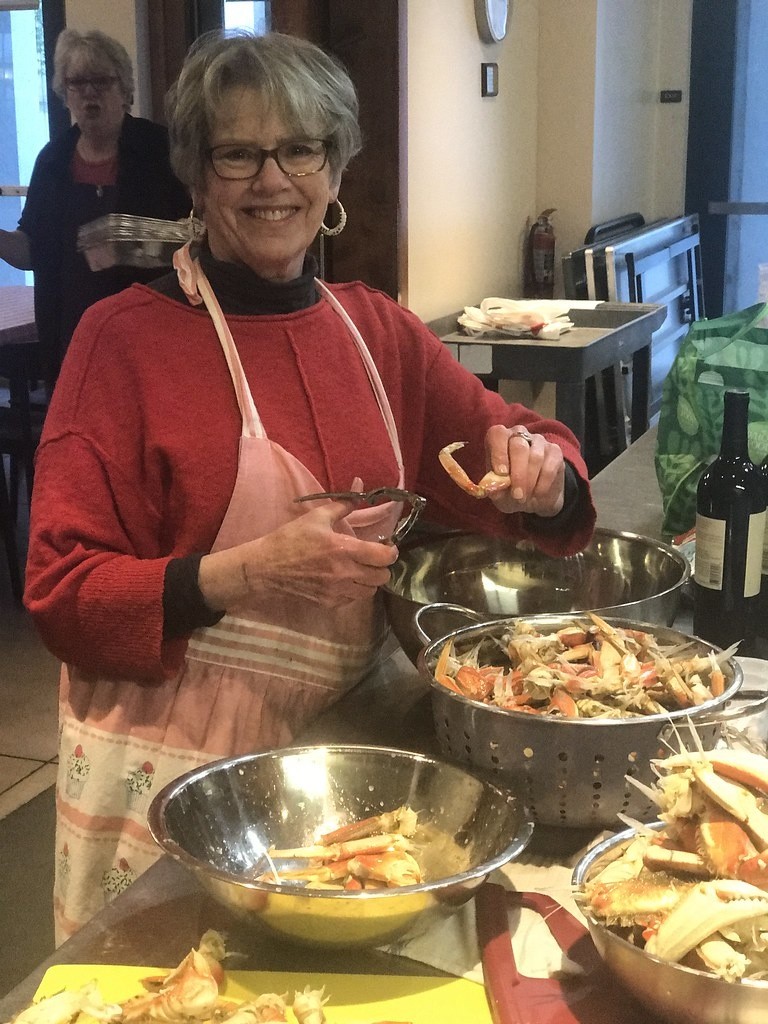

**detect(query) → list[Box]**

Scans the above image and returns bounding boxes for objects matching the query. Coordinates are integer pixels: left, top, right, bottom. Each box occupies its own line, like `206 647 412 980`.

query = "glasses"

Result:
200 138 337 180
62 73 120 92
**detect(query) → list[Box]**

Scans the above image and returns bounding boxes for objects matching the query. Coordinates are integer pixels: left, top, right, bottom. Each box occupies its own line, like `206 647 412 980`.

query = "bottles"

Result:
692 386 768 642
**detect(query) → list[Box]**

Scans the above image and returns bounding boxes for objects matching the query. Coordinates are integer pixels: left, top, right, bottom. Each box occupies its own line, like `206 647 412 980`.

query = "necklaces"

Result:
78 137 118 195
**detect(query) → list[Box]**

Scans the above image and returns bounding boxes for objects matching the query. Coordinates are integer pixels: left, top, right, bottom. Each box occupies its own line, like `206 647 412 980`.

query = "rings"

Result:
508 432 531 446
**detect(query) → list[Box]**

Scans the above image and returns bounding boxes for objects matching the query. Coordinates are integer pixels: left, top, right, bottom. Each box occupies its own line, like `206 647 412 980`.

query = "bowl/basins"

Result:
145 742 535 954
572 820 768 1024
378 523 692 672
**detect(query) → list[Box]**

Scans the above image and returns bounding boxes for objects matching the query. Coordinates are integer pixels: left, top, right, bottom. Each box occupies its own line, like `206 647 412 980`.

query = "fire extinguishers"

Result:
525 207 559 299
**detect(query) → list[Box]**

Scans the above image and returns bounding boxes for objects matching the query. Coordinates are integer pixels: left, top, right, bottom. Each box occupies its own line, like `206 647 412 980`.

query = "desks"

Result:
0 284 49 604
422 297 668 480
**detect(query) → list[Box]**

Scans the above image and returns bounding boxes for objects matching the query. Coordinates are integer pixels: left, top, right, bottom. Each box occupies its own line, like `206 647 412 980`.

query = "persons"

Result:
0 29 192 410
25 30 596 950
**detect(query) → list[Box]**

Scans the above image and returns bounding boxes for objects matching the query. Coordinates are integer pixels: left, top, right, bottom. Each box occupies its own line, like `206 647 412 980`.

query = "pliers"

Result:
292 488 424 542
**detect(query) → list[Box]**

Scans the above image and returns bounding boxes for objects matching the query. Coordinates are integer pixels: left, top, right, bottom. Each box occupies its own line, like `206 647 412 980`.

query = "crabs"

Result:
433 608 741 717
547 716 768 986
260 800 425 887
438 440 511 499
